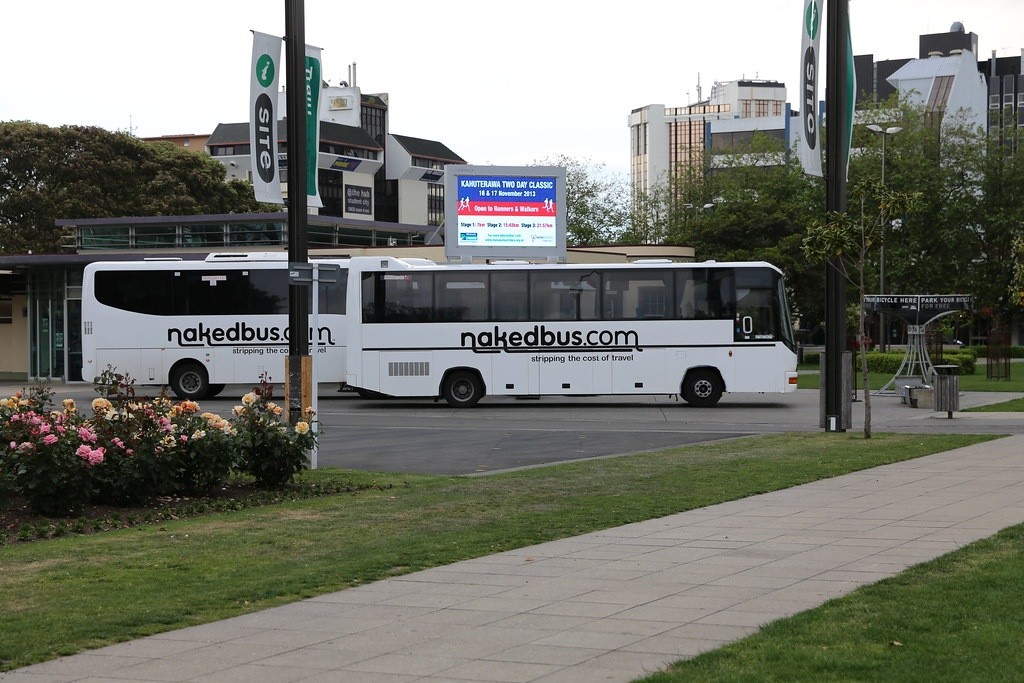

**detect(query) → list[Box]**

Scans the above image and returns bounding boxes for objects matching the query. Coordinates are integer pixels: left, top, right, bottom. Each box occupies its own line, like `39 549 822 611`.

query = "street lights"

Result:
865 124 905 352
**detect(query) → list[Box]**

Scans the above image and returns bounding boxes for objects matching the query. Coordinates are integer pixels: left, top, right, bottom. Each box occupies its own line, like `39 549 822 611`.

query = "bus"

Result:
82 252 802 409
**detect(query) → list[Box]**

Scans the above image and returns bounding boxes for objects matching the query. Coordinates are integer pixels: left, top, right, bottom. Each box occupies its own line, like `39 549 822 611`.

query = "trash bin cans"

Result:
798 346 803 364
819 352 853 429
933 366 960 419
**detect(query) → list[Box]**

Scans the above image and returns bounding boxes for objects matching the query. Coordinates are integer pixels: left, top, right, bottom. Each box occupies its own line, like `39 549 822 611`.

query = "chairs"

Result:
635 307 646 319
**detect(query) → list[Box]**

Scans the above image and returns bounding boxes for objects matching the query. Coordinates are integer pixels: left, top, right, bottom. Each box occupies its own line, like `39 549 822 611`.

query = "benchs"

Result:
904 386 934 410
